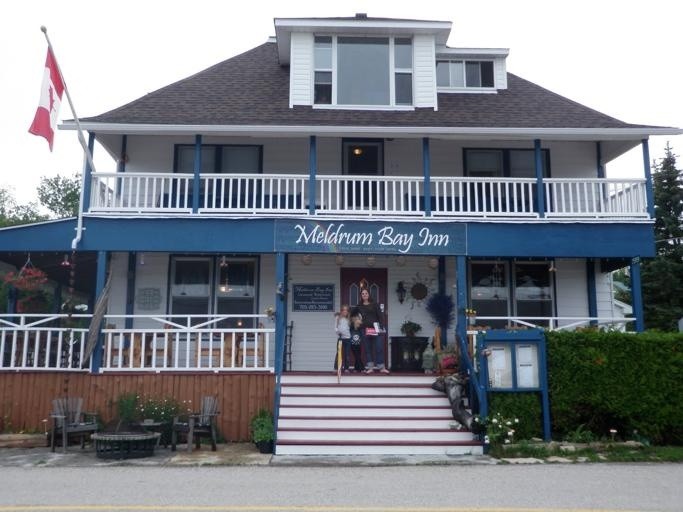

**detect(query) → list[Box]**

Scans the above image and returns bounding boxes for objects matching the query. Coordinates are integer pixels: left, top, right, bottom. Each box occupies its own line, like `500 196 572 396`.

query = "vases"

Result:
494 432 508 445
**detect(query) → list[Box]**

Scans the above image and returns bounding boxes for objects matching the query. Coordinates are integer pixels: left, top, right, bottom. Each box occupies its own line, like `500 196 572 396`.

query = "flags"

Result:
28 46 64 152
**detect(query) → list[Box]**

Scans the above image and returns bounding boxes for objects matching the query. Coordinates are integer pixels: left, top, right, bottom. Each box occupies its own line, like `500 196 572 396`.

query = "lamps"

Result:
396 281 406 304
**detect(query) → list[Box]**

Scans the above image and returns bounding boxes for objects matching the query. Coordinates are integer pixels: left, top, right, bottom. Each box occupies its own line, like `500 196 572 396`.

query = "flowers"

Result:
133 390 192 422
473 412 521 445
1 266 49 292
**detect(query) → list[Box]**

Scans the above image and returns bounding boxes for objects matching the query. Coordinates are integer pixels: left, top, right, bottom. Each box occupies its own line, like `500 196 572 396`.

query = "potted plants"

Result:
399 320 421 336
248 408 275 453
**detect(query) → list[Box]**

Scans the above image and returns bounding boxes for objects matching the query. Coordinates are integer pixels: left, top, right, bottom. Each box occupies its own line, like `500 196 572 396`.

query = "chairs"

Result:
104 323 268 368
47 397 99 454
169 394 221 452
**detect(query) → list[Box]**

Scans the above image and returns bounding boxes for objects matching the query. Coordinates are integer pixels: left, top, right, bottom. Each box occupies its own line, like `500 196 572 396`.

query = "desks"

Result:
87 430 161 459
388 335 429 370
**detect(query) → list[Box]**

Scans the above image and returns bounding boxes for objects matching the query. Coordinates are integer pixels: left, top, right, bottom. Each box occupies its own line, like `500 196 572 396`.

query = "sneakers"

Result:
333 365 391 376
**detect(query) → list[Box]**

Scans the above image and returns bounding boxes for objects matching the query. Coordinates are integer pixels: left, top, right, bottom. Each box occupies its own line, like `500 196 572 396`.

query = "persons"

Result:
335 285 390 374
